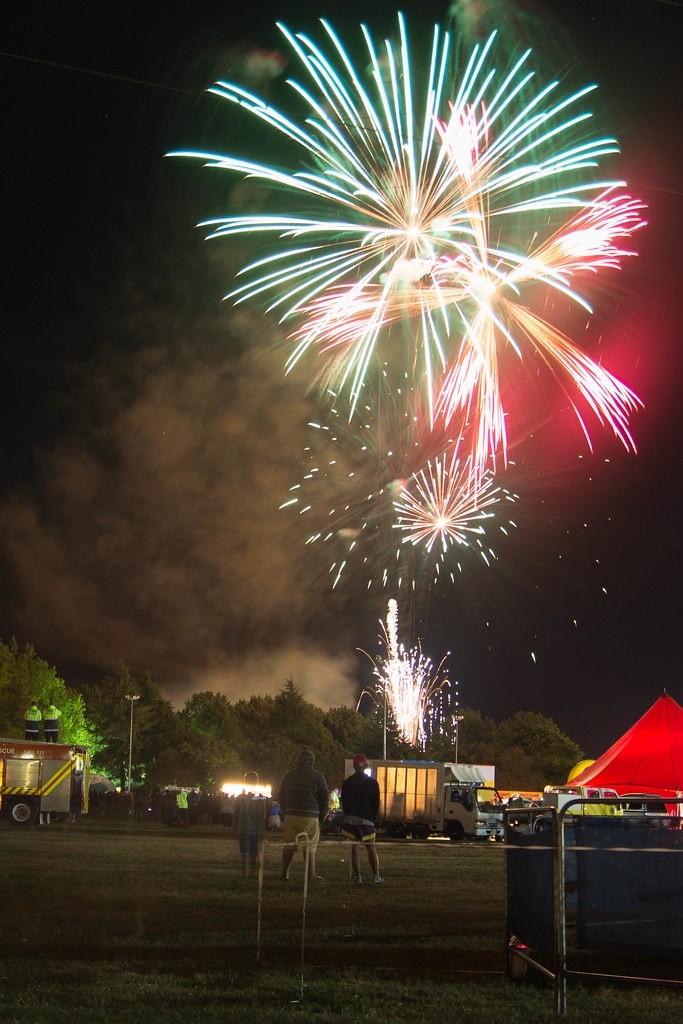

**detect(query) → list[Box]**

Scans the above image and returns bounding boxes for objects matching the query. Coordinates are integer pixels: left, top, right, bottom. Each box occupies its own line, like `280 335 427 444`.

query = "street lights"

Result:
124 693 141 794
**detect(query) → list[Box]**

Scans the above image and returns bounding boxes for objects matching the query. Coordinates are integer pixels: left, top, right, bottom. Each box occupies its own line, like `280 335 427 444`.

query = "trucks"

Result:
344 758 509 843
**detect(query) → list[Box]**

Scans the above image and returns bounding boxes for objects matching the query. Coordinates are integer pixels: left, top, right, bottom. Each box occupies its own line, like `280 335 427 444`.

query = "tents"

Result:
543 688 683 816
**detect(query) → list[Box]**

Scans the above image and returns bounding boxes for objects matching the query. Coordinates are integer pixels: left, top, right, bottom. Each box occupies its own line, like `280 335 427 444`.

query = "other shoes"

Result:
352 875 362 882
373 876 384 883
280 878 289 881
310 876 323 881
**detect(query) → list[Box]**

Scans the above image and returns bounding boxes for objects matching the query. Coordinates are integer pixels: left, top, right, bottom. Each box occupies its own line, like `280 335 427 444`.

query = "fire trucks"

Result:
0 738 91 826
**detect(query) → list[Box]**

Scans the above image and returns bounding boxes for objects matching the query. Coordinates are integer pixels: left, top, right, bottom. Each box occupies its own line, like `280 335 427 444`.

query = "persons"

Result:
278 749 329 880
42 701 62 743
24 701 42 742
232 771 265 882
451 791 465 804
70 771 343 835
339 754 384 884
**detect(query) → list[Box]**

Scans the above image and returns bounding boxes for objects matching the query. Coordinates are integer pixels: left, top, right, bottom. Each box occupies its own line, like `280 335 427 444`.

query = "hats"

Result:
297 751 314 765
353 754 367 766
